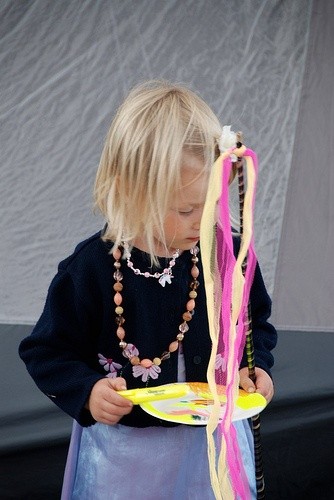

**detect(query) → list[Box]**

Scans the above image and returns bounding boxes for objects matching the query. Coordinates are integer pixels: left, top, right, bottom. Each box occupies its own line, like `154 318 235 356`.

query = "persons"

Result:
18 76 278 500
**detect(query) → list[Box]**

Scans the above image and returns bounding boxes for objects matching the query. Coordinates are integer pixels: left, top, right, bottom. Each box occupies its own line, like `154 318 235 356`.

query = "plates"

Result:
139 382 268 426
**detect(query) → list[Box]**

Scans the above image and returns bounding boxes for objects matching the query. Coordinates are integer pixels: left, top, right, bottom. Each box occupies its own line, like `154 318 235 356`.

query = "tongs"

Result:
116 382 190 404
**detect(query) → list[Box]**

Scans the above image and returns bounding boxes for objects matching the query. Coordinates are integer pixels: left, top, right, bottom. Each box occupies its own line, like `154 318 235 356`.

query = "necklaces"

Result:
113 243 199 382
123 242 180 287
